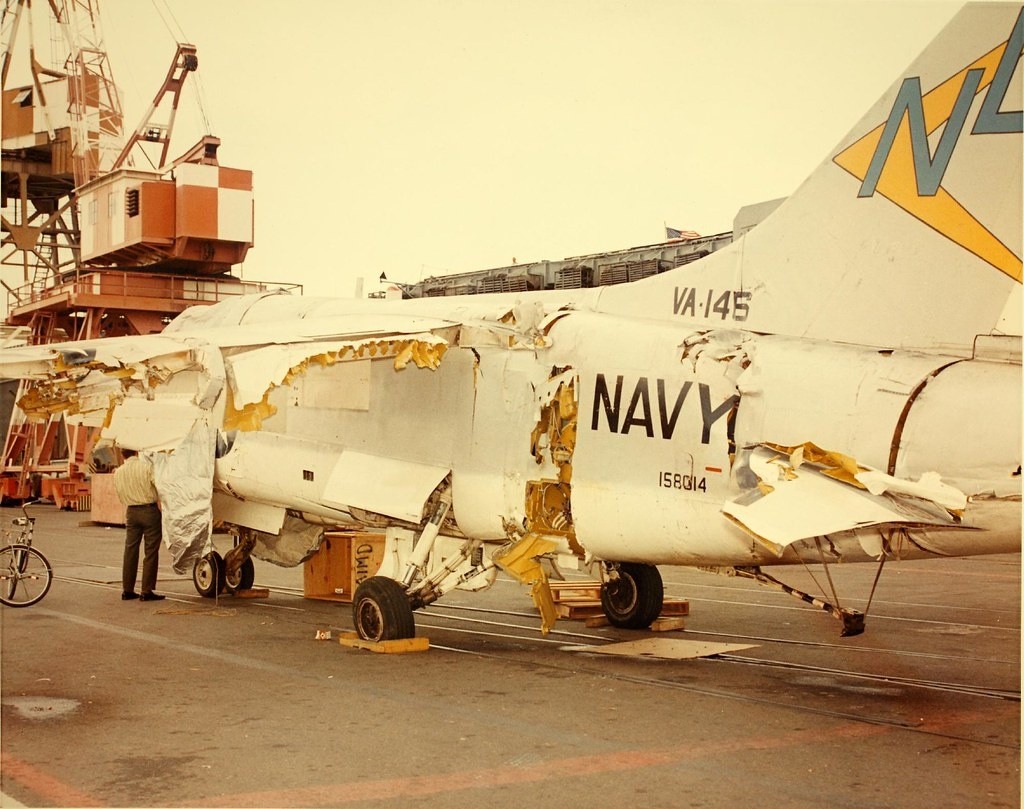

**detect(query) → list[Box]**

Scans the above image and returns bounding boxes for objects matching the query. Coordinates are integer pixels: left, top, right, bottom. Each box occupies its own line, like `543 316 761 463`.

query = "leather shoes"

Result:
122 591 165 601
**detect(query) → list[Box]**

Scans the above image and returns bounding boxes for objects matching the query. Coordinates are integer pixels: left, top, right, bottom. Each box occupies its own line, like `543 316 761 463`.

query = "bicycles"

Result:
0 498 53 608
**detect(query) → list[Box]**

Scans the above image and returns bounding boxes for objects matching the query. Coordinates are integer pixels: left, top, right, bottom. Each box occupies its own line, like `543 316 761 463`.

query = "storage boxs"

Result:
303 532 387 603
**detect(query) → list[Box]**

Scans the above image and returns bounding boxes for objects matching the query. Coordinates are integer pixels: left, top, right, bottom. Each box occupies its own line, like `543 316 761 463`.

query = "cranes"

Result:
1 0 304 499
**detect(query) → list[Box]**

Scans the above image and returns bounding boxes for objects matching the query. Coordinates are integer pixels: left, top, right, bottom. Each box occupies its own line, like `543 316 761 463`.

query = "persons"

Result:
114 448 166 602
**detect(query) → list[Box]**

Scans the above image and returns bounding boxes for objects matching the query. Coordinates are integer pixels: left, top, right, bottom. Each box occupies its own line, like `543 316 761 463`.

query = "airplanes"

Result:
0 1 1024 642
